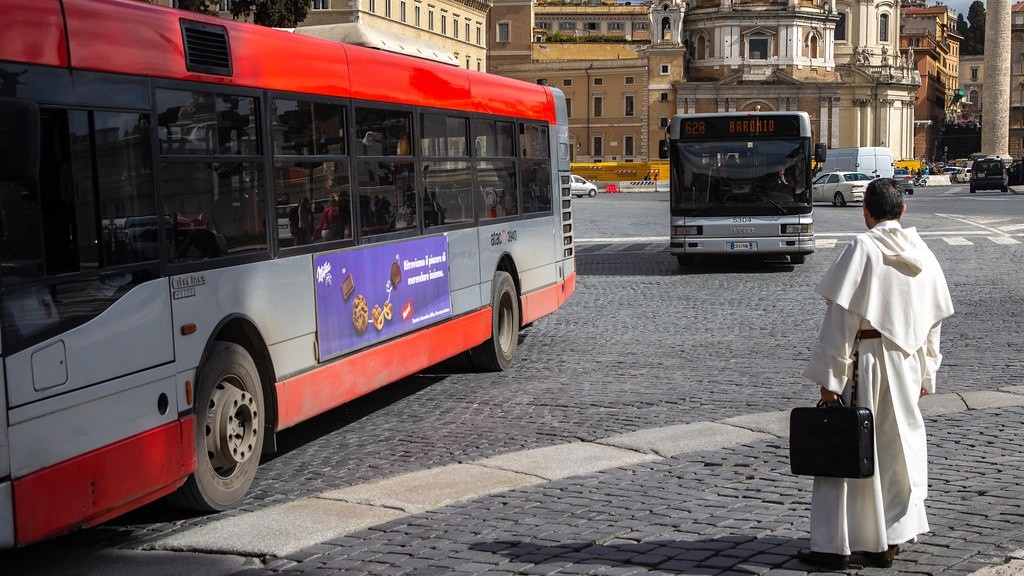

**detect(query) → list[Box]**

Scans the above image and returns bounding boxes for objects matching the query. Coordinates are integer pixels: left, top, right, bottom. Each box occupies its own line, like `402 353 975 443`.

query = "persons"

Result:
769 165 795 185
897 166 929 179
287 192 353 246
786 178 954 567
644 171 651 180
654 174 658 180
853 45 913 68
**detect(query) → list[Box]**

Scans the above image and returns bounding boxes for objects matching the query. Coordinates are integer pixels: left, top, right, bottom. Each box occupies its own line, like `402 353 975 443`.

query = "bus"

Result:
0 1 576 551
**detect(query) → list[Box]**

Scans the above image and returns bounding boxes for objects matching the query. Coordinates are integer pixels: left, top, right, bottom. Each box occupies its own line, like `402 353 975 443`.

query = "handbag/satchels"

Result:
789 394 875 478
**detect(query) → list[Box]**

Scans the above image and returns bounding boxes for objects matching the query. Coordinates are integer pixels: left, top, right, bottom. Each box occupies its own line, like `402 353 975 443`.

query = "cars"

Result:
276 203 300 239
813 171 874 207
570 174 598 198
934 161 972 185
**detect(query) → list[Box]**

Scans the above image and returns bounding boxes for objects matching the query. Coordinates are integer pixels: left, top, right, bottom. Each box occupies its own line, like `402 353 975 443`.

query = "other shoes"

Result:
863 545 900 568
799 548 850 570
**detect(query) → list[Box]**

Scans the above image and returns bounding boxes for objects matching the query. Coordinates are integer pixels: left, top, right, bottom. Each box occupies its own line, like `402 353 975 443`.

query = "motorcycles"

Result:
913 175 927 188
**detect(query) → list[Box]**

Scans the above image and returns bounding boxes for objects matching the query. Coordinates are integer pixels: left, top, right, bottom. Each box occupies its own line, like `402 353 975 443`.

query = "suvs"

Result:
969 157 1008 194
893 167 914 196
658 110 827 266
423 201 447 224
118 216 217 263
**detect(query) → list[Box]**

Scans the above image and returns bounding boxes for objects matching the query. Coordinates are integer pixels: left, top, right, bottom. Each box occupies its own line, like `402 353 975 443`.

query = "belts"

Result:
856 329 881 339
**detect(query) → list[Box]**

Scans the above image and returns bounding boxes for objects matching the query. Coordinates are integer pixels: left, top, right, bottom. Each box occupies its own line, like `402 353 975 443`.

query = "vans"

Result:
812 147 895 179
